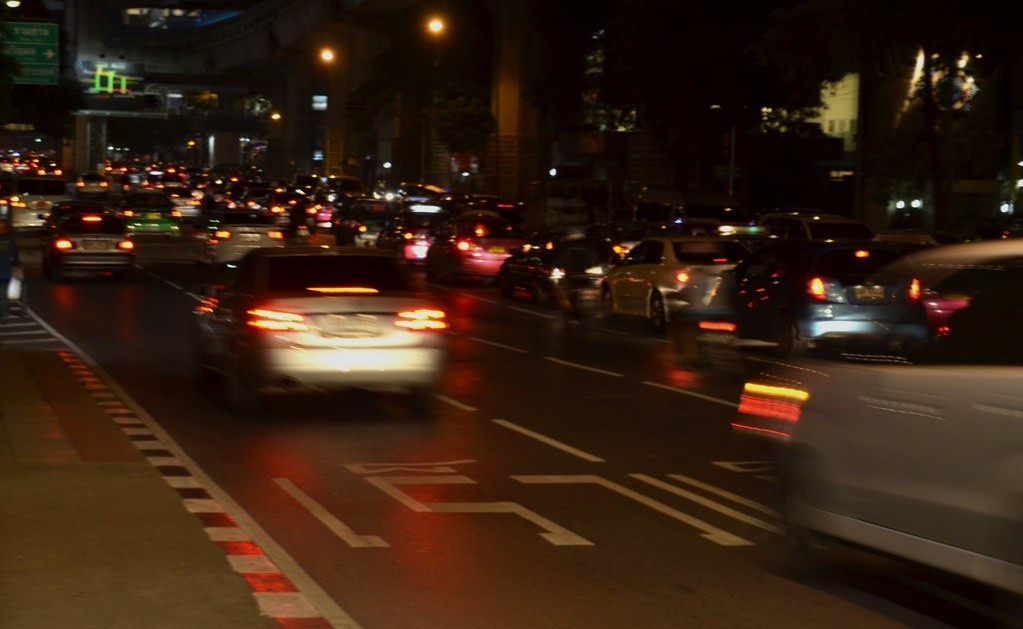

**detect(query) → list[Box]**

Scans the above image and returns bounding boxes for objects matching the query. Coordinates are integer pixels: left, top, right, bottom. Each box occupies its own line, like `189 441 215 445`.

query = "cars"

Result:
771 245 929 373
600 234 749 341
193 246 445 420
0 136 930 320
899 242 1021 360
753 243 1020 602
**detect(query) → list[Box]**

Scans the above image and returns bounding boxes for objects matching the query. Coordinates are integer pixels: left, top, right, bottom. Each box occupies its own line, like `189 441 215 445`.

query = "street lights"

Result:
426 15 449 186
319 45 335 175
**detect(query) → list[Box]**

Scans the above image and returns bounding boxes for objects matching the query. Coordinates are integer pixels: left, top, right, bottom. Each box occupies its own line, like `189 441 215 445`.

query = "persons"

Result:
0 218 23 317
